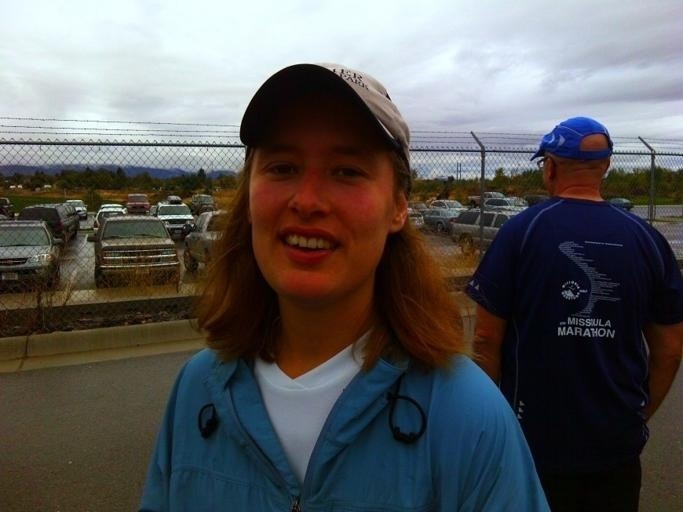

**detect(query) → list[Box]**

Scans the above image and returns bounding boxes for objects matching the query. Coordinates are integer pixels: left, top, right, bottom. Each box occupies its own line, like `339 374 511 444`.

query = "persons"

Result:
471 116 681 510
137 65 549 510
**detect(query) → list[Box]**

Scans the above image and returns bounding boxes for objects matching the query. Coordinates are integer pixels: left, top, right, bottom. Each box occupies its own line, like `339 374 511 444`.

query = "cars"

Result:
0 218 63 292
403 191 548 235
92 204 128 234
607 198 634 211
191 195 215 211
0 198 16 220
66 200 88 220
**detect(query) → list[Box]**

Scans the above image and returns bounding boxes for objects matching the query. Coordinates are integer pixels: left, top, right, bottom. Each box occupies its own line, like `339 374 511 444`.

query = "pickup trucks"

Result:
182 209 229 272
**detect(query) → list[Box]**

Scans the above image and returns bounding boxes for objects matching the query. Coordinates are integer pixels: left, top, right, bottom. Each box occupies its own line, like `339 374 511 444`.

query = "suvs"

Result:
127 193 151 214
17 203 81 244
156 196 196 238
450 210 521 254
86 214 181 288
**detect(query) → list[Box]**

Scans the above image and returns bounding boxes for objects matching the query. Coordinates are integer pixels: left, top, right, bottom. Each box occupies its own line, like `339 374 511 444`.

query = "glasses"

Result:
535 156 555 170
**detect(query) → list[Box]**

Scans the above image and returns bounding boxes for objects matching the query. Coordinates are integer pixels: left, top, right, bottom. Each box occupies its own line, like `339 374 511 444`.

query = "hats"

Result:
240 61 411 168
530 116 614 160
